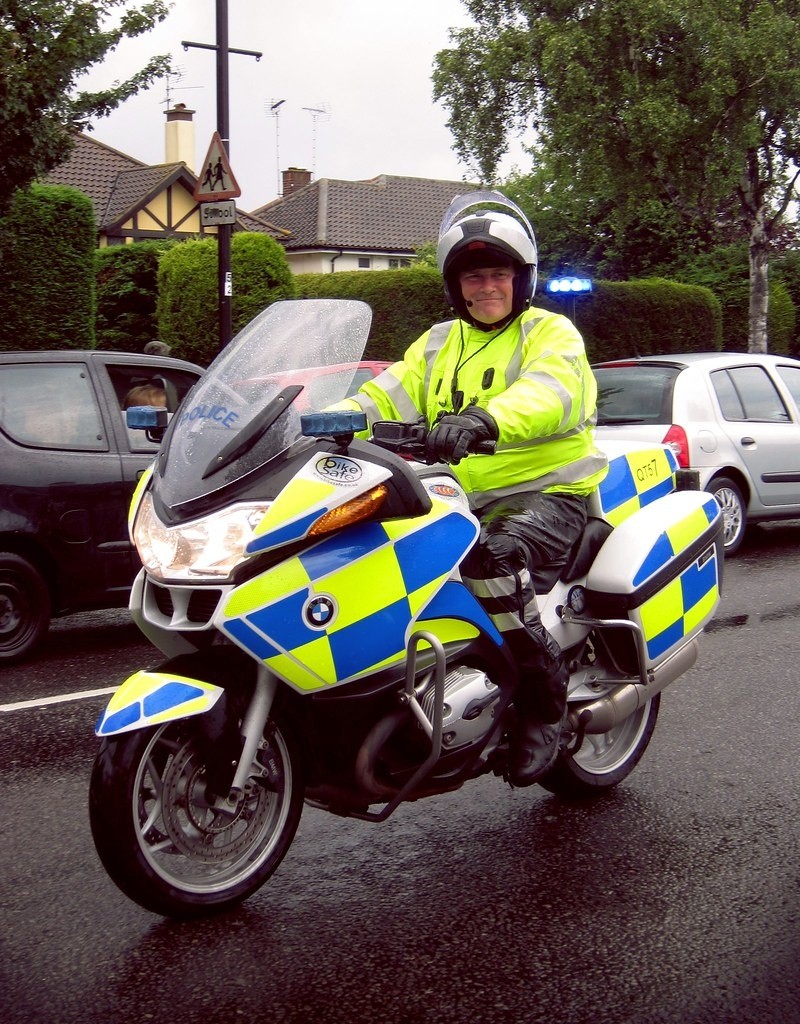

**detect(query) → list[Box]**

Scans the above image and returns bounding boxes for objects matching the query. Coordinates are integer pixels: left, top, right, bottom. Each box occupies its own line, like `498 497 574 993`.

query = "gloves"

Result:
424 405 500 467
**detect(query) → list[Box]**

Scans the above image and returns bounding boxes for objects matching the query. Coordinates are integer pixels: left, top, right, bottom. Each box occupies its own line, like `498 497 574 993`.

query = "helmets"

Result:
436 189 538 312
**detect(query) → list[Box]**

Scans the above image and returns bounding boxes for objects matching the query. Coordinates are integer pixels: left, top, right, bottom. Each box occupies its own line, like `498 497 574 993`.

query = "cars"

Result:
0 350 250 668
589 353 800 558
227 361 395 415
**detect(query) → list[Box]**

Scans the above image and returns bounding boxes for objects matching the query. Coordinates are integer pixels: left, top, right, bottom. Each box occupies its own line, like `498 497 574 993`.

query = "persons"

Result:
120 340 171 411
299 207 602 787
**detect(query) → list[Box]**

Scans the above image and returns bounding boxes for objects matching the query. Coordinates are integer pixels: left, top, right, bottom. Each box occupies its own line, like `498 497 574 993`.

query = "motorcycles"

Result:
87 276 726 920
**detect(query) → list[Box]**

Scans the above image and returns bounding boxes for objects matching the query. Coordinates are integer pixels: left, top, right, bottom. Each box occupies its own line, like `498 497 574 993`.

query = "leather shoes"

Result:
506 703 568 788
